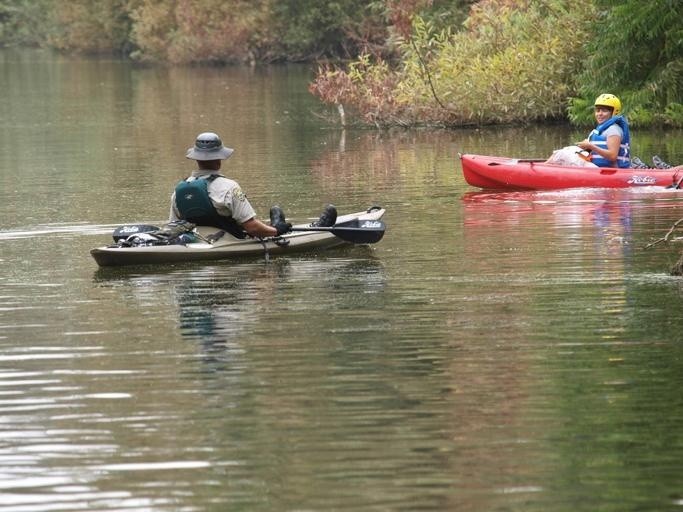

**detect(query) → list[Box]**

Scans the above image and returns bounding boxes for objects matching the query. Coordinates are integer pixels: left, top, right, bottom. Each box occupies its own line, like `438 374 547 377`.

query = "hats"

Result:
185 132 235 162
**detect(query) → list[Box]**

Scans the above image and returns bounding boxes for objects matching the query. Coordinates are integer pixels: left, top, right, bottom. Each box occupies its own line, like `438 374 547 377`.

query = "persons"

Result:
548 93 630 168
168 132 337 248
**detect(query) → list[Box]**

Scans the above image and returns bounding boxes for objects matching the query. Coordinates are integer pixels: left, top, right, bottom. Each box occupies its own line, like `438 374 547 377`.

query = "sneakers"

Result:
309 204 338 228
270 205 286 228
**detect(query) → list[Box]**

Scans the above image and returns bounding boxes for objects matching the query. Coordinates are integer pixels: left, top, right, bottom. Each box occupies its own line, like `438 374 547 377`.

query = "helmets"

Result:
594 93 622 119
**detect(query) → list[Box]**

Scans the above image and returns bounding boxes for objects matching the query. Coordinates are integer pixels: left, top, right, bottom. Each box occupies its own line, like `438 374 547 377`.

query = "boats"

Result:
458 153 683 191
90 205 386 272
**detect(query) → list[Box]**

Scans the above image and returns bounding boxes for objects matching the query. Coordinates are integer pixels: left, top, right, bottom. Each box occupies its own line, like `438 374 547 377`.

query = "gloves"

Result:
275 222 292 235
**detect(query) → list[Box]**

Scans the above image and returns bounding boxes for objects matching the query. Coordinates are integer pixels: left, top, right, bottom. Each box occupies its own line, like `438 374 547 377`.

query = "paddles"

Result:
289 218 386 243
113 224 161 244
